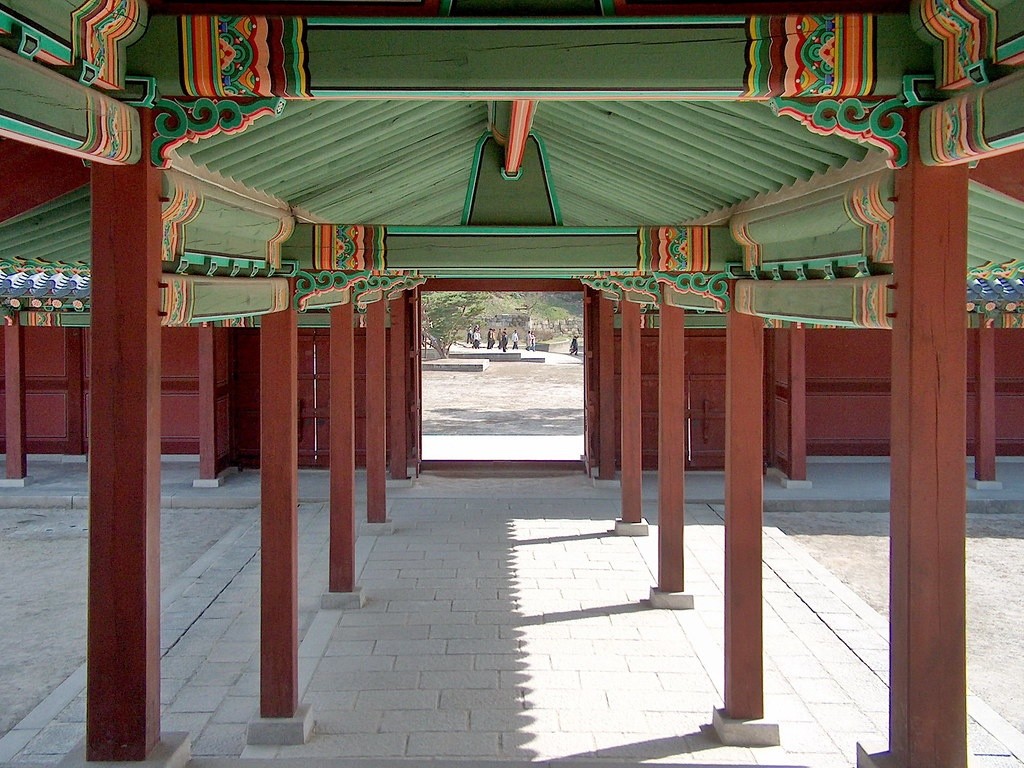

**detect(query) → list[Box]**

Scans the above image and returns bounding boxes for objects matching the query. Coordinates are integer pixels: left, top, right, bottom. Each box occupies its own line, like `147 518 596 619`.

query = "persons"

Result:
569 334 580 356
466 323 537 352
